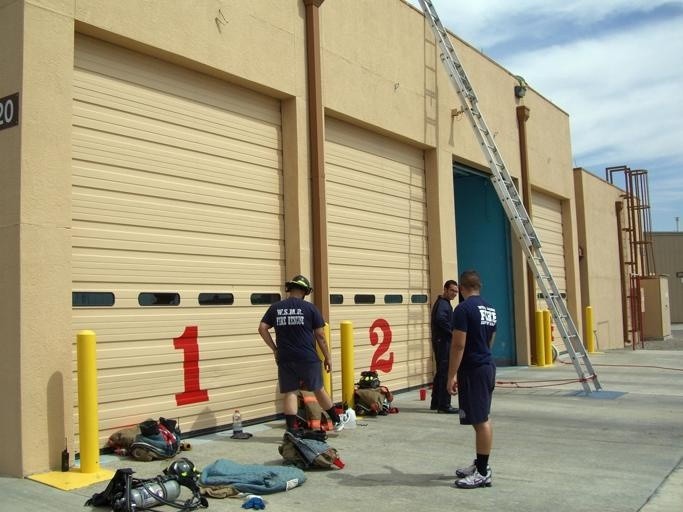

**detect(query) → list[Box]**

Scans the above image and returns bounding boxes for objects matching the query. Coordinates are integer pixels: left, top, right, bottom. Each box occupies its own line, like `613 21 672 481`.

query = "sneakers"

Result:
333 413 350 432
455 468 491 488
456 459 489 478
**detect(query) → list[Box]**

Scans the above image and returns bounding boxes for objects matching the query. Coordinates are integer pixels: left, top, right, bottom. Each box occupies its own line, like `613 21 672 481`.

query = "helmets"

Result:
285 275 310 295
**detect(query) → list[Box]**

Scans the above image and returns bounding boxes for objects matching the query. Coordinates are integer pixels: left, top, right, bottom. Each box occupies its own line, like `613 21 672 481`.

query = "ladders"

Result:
417 0 602 395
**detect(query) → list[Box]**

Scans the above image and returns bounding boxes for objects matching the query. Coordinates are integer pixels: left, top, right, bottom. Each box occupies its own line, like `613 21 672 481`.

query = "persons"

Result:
258 274 351 434
430 280 460 413
447 270 497 489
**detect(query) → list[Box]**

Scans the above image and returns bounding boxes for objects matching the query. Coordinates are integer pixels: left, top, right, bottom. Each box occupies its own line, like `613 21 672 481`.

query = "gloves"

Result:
242 495 265 510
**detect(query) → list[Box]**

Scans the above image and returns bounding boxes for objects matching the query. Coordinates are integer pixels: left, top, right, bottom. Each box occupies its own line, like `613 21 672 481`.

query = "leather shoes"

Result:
431 404 460 413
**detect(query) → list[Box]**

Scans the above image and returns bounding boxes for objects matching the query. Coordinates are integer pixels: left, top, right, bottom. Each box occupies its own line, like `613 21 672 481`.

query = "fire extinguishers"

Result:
551 324 555 341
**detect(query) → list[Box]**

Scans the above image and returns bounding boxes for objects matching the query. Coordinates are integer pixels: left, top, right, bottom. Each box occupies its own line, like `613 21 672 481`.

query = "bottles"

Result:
419 385 426 400
62 450 69 472
231 409 242 434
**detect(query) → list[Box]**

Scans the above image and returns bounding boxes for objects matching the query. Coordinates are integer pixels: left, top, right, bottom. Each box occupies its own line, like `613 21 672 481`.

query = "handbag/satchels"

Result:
279 431 344 471
